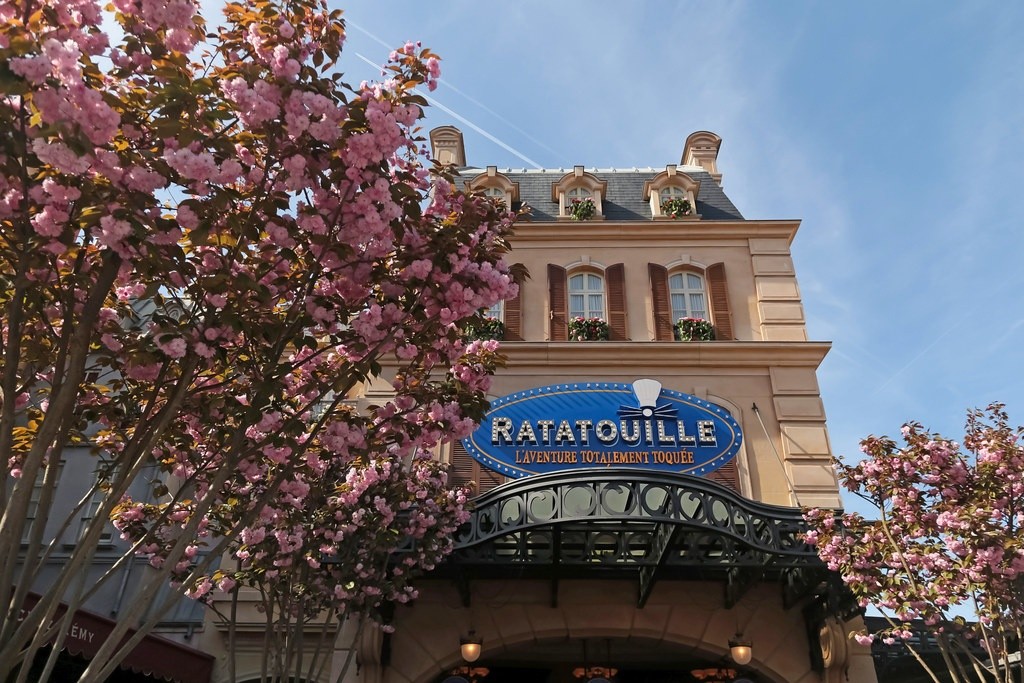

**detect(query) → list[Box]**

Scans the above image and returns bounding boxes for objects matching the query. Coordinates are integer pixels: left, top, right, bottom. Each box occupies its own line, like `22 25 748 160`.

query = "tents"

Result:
4 584 216 683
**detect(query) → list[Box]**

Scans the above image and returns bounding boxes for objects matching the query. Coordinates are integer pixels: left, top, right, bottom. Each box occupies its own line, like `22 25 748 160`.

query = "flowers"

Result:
569 316 608 342
470 317 503 342
569 197 595 221
678 317 713 342
662 197 691 219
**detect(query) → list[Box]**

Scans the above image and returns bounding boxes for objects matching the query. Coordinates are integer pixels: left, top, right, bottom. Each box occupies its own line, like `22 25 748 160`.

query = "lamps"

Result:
460 579 483 662
728 569 753 665
